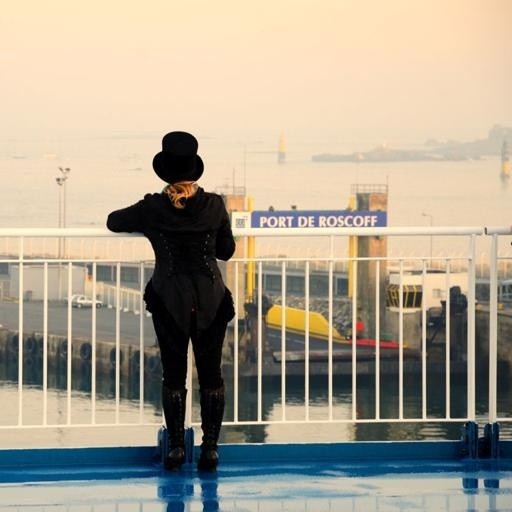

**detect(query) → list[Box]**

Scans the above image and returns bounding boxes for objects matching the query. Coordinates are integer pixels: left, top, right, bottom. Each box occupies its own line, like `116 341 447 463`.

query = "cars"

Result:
63 294 103 309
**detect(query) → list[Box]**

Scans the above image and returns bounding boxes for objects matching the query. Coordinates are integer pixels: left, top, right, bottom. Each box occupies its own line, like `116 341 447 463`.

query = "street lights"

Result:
54 166 71 258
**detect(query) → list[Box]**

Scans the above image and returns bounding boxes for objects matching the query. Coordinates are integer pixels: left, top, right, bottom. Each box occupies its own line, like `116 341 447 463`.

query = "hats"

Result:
153 131 203 185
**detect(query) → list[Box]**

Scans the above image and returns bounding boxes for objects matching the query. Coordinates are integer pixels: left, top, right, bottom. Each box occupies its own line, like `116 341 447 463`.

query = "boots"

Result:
160 386 187 473
197 386 225 472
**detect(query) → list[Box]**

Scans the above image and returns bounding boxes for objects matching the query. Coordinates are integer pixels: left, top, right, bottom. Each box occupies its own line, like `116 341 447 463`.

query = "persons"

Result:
106 132 237 474
